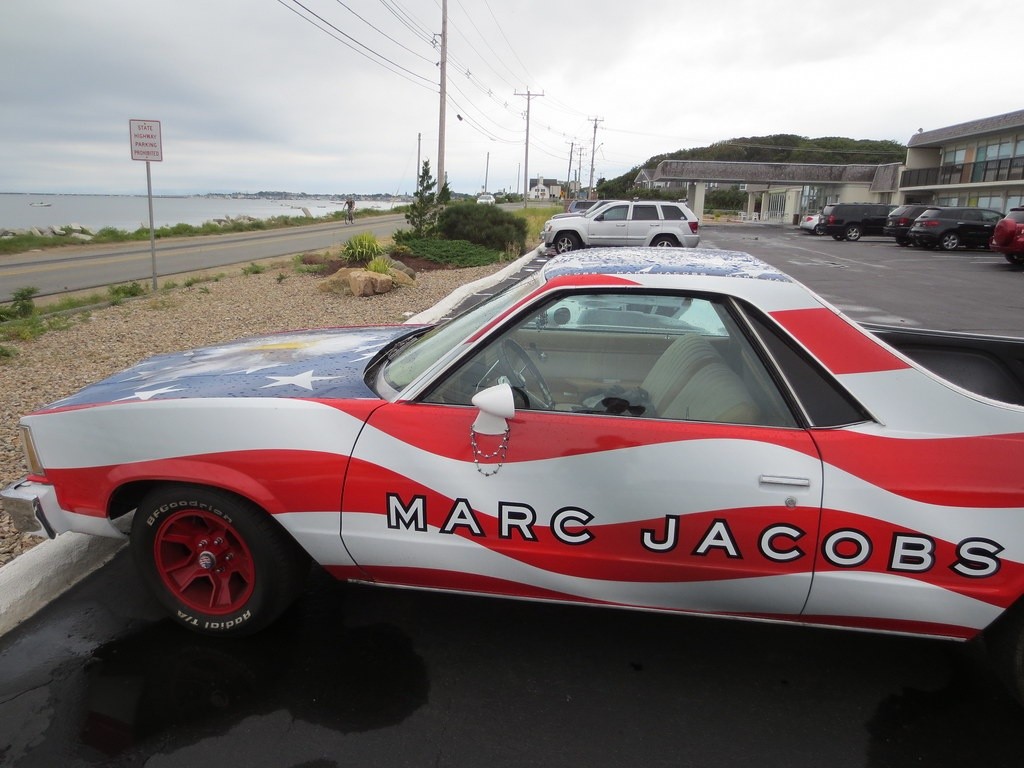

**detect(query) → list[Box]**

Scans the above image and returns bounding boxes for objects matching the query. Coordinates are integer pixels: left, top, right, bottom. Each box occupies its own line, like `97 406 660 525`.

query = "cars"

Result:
475 195 497 206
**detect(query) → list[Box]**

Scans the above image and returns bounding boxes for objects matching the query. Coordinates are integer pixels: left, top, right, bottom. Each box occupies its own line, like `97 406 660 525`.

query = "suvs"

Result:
884 204 1005 251
541 198 700 254
988 204 1024 270
799 202 899 241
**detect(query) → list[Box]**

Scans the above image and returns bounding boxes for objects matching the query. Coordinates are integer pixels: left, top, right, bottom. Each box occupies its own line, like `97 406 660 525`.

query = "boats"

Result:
29 201 52 207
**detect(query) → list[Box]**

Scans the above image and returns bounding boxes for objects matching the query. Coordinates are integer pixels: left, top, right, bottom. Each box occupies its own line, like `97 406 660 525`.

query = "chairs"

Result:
640 332 762 424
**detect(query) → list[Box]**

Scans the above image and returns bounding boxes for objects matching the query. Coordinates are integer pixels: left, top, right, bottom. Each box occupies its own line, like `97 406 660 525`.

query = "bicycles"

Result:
344 209 355 225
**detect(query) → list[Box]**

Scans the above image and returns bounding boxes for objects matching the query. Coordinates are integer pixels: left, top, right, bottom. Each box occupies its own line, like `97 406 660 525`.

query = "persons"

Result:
343 196 355 221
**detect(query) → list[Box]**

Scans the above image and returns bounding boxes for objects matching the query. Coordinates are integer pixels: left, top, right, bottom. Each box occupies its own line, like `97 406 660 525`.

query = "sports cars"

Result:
1 246 1024 651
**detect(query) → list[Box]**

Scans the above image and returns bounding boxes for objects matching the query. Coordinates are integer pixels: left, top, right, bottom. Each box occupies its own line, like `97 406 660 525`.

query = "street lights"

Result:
588 143 604 200
